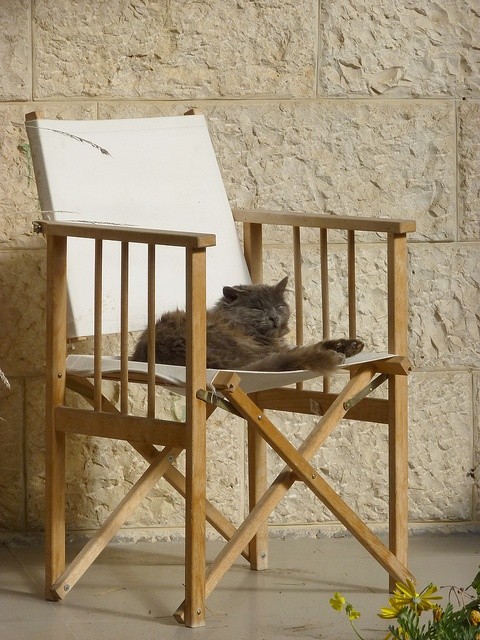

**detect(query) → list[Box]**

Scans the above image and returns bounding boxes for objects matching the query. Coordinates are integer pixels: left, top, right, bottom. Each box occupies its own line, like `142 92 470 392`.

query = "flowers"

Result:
330 581 479 639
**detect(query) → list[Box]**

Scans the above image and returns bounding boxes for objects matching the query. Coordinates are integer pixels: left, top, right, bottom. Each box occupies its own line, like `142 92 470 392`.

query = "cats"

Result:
129 276 364 374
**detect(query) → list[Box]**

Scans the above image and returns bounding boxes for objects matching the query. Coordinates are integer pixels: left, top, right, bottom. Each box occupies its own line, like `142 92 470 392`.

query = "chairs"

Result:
26 103 417 629
